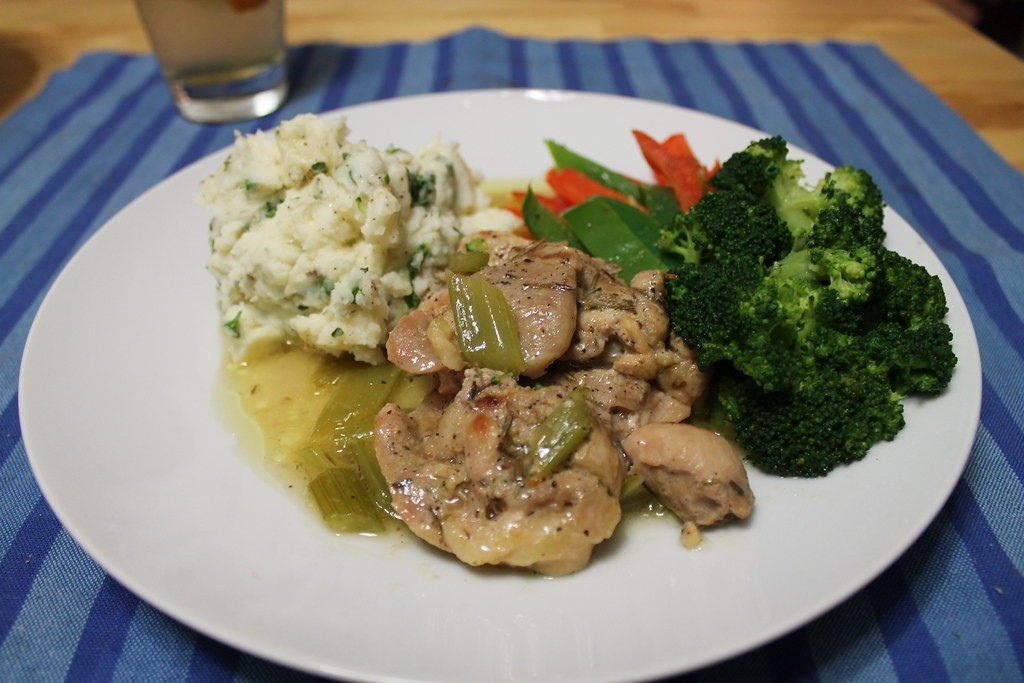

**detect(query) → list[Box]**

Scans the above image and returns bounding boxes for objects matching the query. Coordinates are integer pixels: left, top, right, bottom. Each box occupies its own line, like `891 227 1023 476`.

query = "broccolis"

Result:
653 134 958 479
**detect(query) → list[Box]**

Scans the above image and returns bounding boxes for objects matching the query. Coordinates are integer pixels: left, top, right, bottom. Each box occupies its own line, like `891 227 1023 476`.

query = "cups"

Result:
129 0 291 124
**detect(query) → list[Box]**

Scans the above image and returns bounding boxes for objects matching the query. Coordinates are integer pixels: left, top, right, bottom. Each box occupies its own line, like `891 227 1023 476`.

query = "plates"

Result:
17 87 983 683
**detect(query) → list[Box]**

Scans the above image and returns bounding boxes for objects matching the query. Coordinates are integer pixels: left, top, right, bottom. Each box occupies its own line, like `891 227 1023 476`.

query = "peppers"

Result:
507 129 719 283
